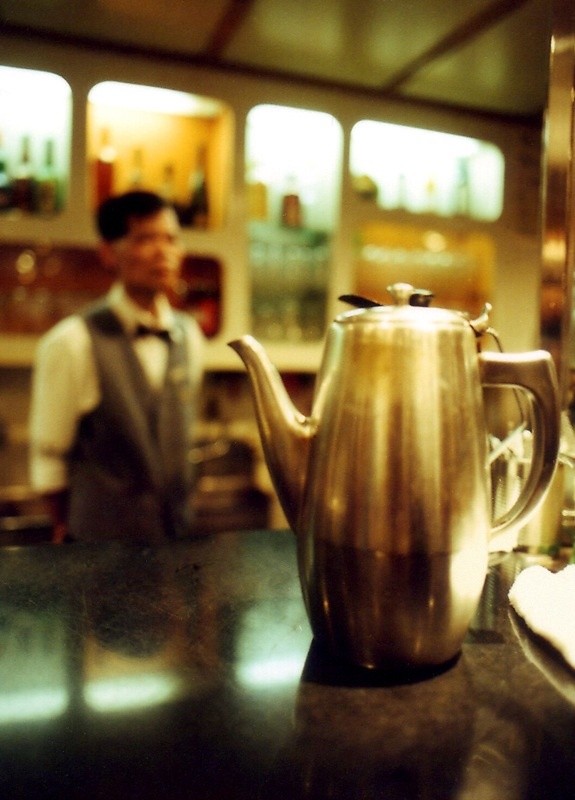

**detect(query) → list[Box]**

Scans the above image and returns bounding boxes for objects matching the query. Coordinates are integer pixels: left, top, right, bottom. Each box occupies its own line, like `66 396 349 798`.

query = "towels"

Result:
507 564 575 669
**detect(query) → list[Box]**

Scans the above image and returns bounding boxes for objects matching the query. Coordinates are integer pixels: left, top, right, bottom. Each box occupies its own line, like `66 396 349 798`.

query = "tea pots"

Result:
226 282 563 681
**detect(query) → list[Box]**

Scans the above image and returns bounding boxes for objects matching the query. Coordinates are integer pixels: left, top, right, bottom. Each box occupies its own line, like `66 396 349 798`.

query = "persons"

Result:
27 190 207 547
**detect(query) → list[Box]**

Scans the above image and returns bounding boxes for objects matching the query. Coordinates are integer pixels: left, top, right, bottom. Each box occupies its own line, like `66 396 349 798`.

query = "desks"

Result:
0 520 575 800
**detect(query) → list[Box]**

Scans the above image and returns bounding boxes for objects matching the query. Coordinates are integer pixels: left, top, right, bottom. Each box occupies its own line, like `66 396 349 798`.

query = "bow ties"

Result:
135 323 172 345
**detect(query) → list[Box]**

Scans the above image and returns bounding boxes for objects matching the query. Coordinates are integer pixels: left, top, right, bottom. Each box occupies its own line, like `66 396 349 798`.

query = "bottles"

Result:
0 134 211 228
